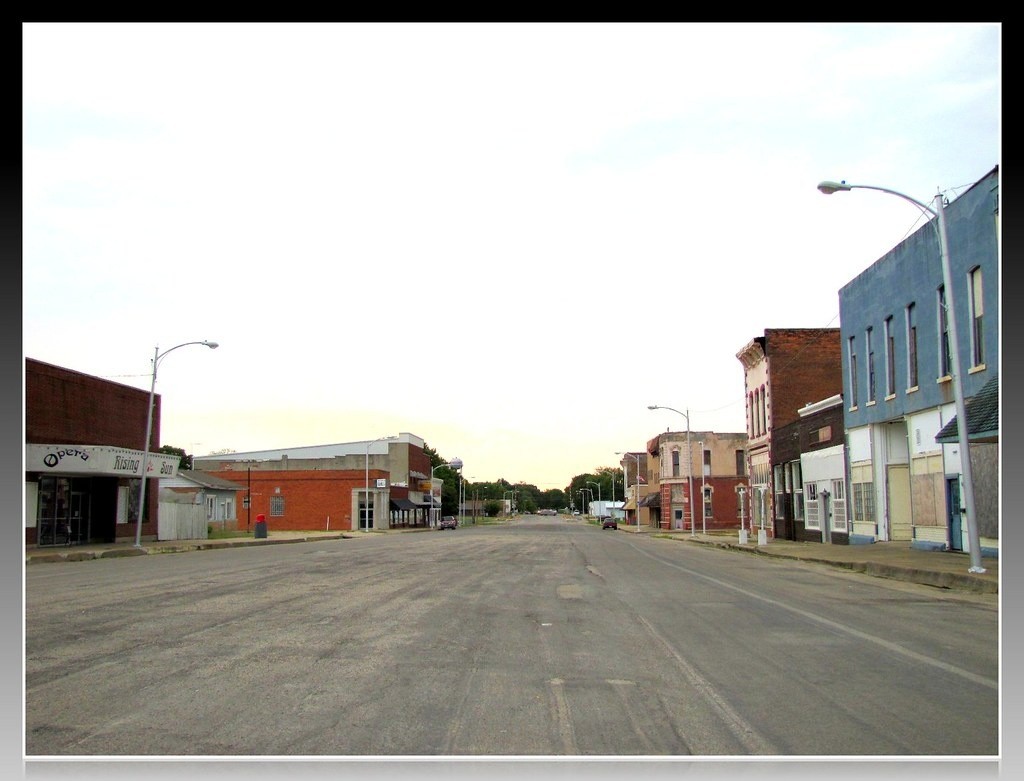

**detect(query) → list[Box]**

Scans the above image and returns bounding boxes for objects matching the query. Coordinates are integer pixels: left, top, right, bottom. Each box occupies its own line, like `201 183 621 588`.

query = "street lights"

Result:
459 477 475 526
580 489 595 519
615 451 640 529
135 341 219 545
648 406 696 538
365 435 395 532
431 463 462 530
576 491 584 515
817 182 982 573
587 481 601 525
597 470 614 518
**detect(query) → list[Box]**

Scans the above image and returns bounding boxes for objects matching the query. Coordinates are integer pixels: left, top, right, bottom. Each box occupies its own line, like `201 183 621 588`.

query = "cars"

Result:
603 518 617 530
538 509 557 516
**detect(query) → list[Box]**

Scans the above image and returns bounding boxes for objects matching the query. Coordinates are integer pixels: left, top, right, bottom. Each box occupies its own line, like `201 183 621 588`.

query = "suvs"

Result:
574 510 579 515
441 516 456 530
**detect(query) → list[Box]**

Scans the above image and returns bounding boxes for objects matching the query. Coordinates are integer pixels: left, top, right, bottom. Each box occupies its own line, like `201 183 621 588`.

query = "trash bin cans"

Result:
254 514 268 539
